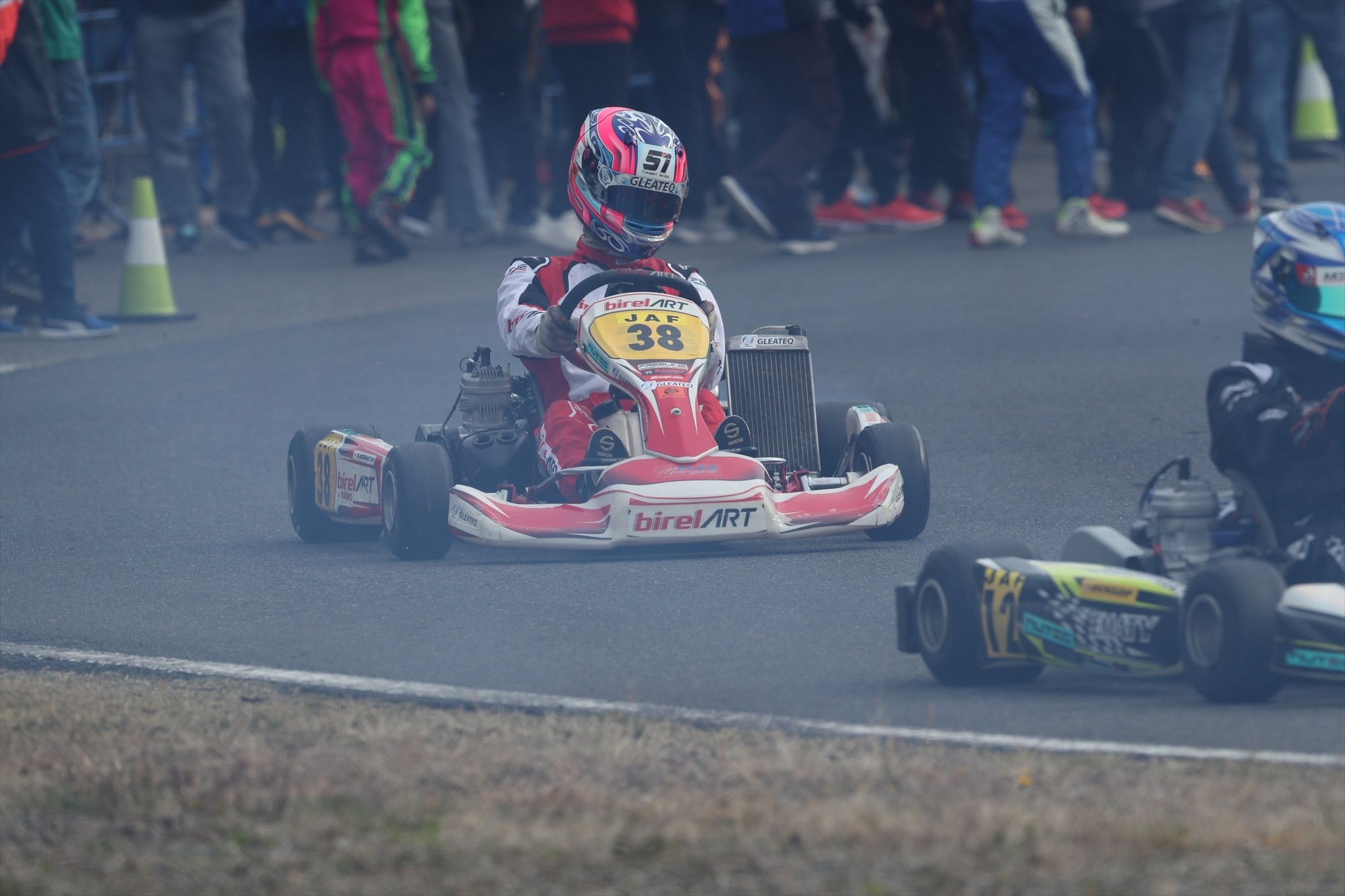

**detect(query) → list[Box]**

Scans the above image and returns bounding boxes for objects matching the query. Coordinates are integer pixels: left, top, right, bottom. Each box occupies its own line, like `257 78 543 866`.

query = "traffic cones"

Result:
92 177 200 325
1286 33 1341 158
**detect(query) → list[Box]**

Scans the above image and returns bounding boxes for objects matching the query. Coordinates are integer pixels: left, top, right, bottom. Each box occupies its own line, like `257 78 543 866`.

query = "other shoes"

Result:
508 207 586 253
906 190 963 218
1259 187 1314 209
37 307 119 338
969 205 1024 250
674 213 736 244
176 222 198 246
255 208 323 242
777 224 836 255
1235 201 1262 225
1088 192 1129 220
0 319 29 339
587 428 628 485
1054 196 1131 239
811 191 949 232
356 209 410 262
714 414 753 458
1155 194 1222 235
957 192 1025 229
219 212 259 243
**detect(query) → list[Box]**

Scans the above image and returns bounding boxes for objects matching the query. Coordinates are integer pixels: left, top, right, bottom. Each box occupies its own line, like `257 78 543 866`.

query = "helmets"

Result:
1244 200 1344 362
565 107 688 259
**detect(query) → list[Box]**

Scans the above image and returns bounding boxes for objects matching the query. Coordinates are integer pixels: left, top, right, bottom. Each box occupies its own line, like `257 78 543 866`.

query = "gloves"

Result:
534 302 579 358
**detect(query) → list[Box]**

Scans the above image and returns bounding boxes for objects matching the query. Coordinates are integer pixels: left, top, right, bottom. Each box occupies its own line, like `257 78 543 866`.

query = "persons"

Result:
0 0 1345 338
1207 202 1345 586
497 106 752 505
